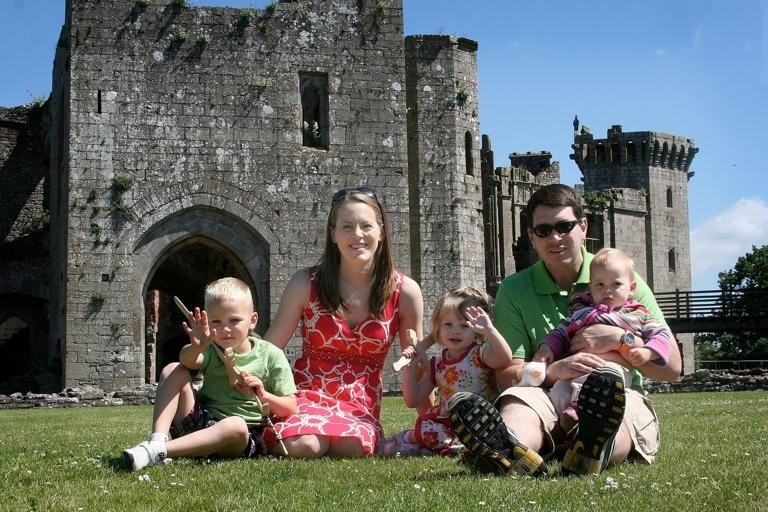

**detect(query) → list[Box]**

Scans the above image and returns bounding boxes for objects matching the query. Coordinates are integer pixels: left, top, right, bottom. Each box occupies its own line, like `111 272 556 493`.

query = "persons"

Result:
382 285 515 460
263 188 434 460
532 247 672 433
445 183 684 481
120 276 297 472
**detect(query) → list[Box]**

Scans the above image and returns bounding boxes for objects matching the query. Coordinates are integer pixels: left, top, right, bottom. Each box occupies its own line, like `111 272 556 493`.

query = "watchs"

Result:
617 328 636 354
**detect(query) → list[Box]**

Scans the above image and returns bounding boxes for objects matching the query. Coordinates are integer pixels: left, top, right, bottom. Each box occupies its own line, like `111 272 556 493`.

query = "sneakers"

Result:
556 364 625 477
120 433 168 473
384 429 420 456
446 392 548 478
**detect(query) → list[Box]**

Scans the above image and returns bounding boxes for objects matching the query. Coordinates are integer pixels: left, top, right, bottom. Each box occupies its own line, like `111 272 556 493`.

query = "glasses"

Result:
332 187 380 200
531 218 582 239
